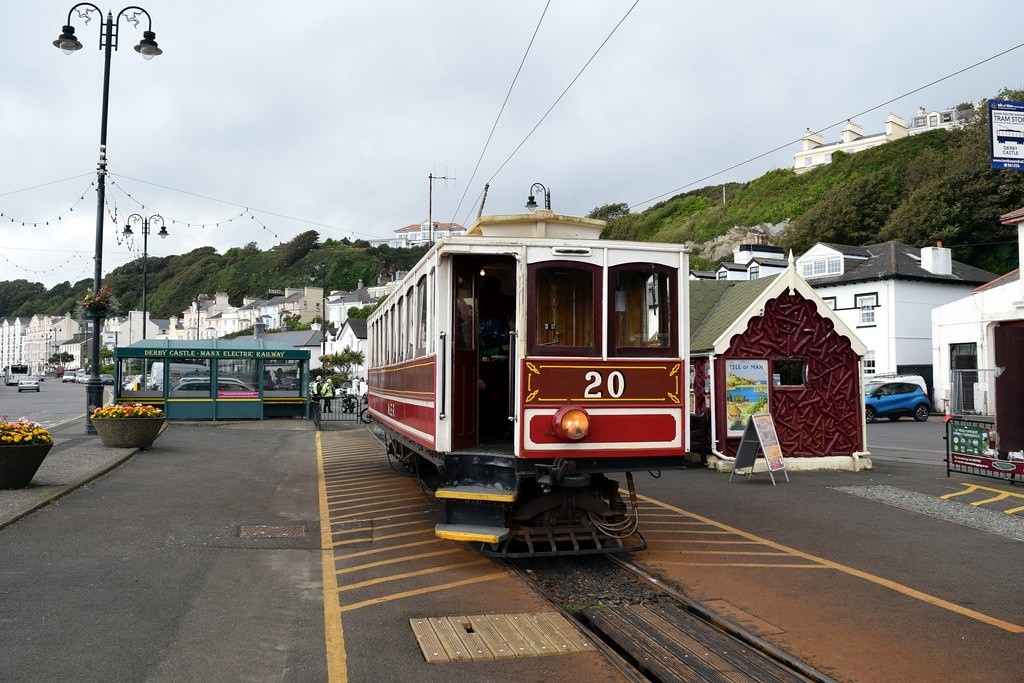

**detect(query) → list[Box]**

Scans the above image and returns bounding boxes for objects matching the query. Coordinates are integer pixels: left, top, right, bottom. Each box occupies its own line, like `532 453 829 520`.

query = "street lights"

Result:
309 263 327 383
524 181 552 212
189 299 200 340
122 214 170 388
49 325 63 353
122 312 132 345
41 332 52 369
80 320 88 375
50 2 164 435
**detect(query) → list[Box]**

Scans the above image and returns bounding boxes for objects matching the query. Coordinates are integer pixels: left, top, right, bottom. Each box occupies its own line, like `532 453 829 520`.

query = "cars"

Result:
61 362 257 396
17 376 41 392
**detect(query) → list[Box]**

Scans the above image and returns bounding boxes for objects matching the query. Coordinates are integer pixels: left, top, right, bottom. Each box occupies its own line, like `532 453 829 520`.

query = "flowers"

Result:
82 285 113 311
0 416 52 442
90 400 165 419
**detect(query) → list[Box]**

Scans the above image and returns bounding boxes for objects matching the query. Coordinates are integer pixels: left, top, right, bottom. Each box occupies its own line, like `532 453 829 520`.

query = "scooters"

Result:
360 407 372 424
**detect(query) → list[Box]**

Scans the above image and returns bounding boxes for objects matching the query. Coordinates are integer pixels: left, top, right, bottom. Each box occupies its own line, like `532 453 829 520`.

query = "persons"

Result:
322 379 334 413
314 376 323 402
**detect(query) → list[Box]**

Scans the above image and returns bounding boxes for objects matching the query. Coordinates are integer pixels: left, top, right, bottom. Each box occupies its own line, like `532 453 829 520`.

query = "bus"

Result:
2 364 31 385
365 234 694 562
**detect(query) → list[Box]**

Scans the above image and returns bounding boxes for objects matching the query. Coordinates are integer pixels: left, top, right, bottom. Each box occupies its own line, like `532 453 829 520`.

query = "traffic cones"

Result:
942 401 956 423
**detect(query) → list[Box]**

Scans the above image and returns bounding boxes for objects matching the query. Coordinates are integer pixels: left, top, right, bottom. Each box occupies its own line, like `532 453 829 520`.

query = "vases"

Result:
87 308 108 319
89 417 166 447
0 442 54 491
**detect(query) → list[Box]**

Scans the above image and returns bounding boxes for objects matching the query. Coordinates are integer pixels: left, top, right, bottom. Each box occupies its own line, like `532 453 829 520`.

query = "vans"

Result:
868 372 928 395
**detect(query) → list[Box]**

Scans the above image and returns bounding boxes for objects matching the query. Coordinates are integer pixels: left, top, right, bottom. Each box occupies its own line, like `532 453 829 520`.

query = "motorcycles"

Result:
338 382 356 414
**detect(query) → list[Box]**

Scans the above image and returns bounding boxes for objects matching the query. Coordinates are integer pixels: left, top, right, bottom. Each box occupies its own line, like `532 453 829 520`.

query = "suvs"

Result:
863 382 932 425
